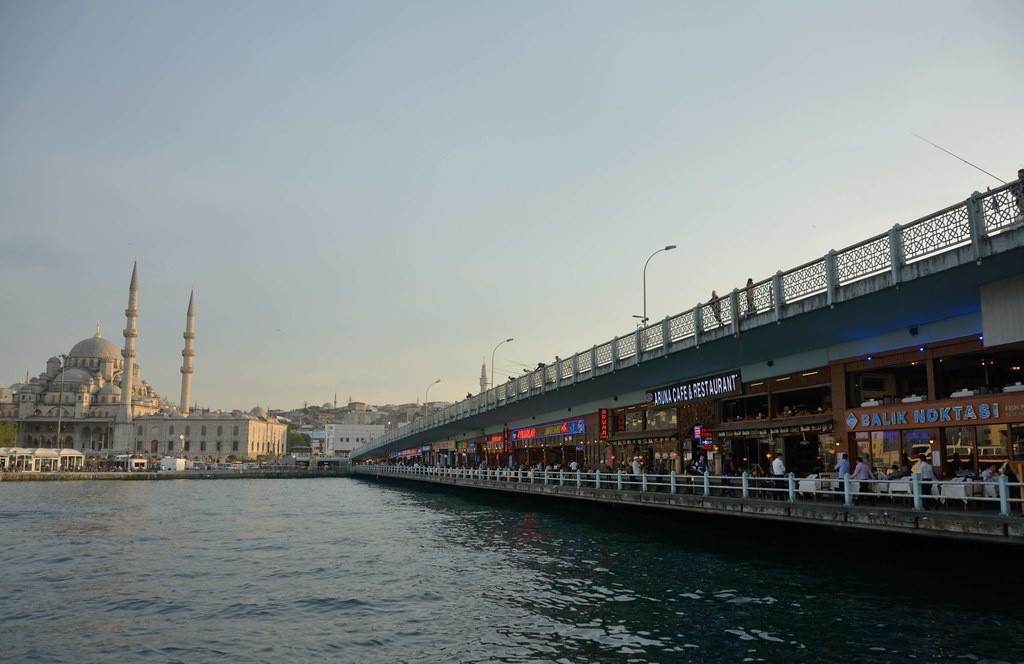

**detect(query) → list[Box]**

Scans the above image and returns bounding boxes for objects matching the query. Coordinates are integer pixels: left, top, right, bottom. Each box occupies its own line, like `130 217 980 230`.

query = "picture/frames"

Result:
862 378 886 391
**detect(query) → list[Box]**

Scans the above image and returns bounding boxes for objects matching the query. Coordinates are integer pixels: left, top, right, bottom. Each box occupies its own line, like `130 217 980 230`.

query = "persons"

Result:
386 458 703 497
693 452 999 500
639 327 650 351
708 290 725 325
507 361 549 395
745 278 757 313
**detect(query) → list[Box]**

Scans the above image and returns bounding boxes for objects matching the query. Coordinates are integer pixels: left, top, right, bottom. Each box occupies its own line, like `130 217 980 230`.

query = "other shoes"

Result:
935 505 941 510
924 506 930 511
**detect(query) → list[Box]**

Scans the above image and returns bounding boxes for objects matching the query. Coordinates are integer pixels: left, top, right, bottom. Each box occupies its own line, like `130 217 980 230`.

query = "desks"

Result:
534 473 728 497
871 483 889 507
940 480 983 512
733 419 742 421
985 486 1000 513
798 481 839 502
860 401 879 407
950 391 975 398
1002 385 1024 393
901 396 922 404
888 483 913 509
851 482 871 506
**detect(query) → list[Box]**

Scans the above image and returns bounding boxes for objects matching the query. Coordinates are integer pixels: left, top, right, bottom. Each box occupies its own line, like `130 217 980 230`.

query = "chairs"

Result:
795 410 804 416
968 477 984 511
727 417 736 422
817 474 830 501
776 412 783 418
742 416 750 420
979 383 990 395
750 415 756 420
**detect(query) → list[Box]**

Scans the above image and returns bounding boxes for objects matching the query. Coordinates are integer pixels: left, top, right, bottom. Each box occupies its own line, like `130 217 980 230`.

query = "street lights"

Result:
425 379 441 417
491 338 515 389
644 245 677 328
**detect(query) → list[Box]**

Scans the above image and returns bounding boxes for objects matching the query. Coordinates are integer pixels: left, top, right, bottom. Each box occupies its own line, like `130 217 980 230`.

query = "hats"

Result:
634 456 638 460
